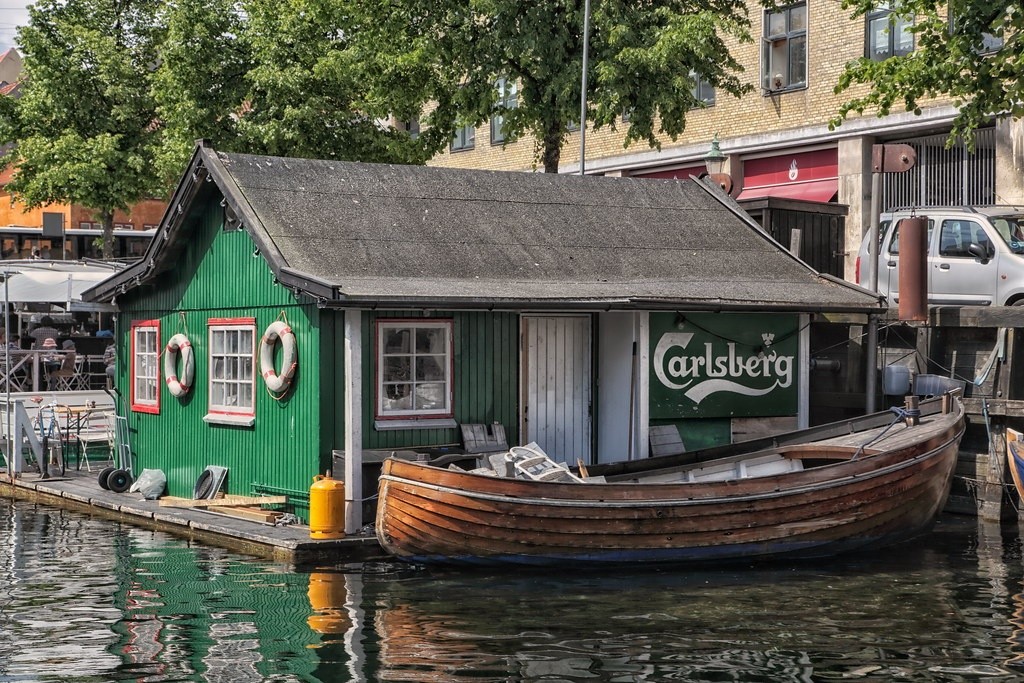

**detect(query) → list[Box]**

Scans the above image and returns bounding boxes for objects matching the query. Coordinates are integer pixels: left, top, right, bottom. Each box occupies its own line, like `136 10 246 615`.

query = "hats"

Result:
62 340 75 350
42 339 57 347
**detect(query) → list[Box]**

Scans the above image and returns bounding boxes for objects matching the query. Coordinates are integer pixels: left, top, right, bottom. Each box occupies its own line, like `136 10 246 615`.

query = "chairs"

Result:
50 355 85 390
87 355 110 390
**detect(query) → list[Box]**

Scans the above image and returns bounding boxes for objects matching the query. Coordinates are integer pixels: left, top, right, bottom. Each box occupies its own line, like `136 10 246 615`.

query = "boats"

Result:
370 386 966 568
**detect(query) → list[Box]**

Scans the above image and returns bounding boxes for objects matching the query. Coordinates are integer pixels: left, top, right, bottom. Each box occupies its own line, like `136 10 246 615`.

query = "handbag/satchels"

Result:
505 446 586 484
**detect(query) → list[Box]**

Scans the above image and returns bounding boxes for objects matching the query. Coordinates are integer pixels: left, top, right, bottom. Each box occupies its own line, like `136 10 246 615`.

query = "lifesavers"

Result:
260 320 297 392
164 334 194 397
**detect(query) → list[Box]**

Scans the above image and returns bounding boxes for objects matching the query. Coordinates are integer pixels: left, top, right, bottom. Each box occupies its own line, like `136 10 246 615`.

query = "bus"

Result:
2 225 162 258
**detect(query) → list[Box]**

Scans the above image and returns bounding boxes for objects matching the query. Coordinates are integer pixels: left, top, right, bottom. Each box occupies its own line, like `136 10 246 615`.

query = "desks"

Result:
19 333 111 359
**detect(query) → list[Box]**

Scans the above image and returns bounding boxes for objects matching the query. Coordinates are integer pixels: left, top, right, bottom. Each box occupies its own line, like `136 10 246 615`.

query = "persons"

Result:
8 335 32 385
103 344 115 376
0 327 12 393
1 241 145 263
30 316 62 390
50 340 75 392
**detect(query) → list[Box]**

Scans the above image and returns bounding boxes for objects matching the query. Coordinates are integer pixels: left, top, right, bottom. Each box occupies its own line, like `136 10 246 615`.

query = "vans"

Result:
855 203 1024 306
